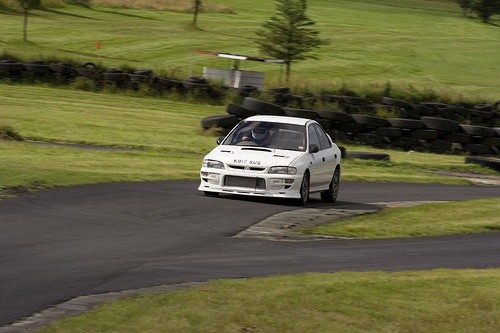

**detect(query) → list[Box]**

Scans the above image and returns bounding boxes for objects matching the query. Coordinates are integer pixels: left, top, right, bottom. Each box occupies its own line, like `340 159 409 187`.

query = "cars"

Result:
198 114 342 207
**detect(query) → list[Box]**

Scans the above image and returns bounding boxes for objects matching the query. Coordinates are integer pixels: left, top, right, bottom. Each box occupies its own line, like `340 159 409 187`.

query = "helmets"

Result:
252 127 269 140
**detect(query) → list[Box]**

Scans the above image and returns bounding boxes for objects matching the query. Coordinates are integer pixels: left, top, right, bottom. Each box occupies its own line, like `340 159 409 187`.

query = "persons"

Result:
242 128 271 146
